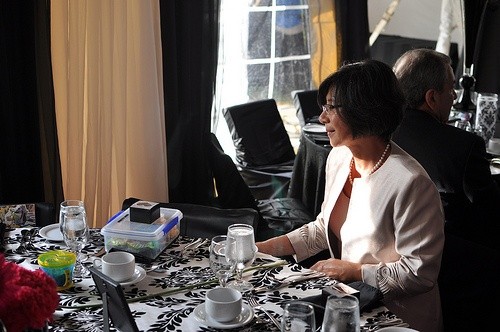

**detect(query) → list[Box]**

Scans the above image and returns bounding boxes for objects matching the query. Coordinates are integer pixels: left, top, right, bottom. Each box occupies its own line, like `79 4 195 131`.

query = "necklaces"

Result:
348 143 390 185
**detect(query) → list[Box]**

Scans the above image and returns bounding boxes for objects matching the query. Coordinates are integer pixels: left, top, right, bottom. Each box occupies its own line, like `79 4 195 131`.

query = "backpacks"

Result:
183 128 269 229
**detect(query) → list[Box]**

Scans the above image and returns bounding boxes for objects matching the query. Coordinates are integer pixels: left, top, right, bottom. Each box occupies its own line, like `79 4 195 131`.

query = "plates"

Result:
192 301 254 329
38 223 63 242
302 124 327 133
97 265 146 286
486 150 500 155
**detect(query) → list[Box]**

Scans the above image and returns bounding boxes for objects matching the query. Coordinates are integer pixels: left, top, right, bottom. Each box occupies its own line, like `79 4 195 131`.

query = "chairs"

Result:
121 198 259 243
210 88 323 243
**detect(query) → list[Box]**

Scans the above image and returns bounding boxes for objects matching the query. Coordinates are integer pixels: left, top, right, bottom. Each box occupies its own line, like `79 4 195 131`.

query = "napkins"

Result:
279 281 385 328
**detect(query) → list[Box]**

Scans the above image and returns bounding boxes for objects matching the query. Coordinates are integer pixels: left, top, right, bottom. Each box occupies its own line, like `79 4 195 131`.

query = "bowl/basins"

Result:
374 326 419 332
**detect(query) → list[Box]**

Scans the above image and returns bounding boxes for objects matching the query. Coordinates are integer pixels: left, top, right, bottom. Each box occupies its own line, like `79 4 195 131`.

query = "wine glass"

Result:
59 200 90 277
208 235 236 288
225 224 256 293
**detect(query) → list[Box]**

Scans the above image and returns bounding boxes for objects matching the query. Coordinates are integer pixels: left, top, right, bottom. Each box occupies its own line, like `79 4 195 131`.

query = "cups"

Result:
37 249 77 290
92 251 135 283
475 92 498 146
280 302 316 332
454 120 471 132
488 138 500 153
320 294 361 332
205 287 242 322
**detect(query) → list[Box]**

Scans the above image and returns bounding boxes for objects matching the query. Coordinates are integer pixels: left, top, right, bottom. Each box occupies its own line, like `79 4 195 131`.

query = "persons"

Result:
253 59 444 332
391 49 500 332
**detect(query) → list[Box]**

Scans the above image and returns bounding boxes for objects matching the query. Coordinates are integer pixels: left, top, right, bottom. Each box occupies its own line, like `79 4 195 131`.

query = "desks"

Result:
288 115 333 221
1 228 409 332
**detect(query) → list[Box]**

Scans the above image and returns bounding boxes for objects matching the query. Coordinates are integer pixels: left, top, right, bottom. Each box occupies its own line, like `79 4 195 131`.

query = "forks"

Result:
245 293 288 332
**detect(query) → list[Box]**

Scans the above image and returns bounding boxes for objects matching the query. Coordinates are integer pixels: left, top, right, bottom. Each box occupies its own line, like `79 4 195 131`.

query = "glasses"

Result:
322 104 341 114
